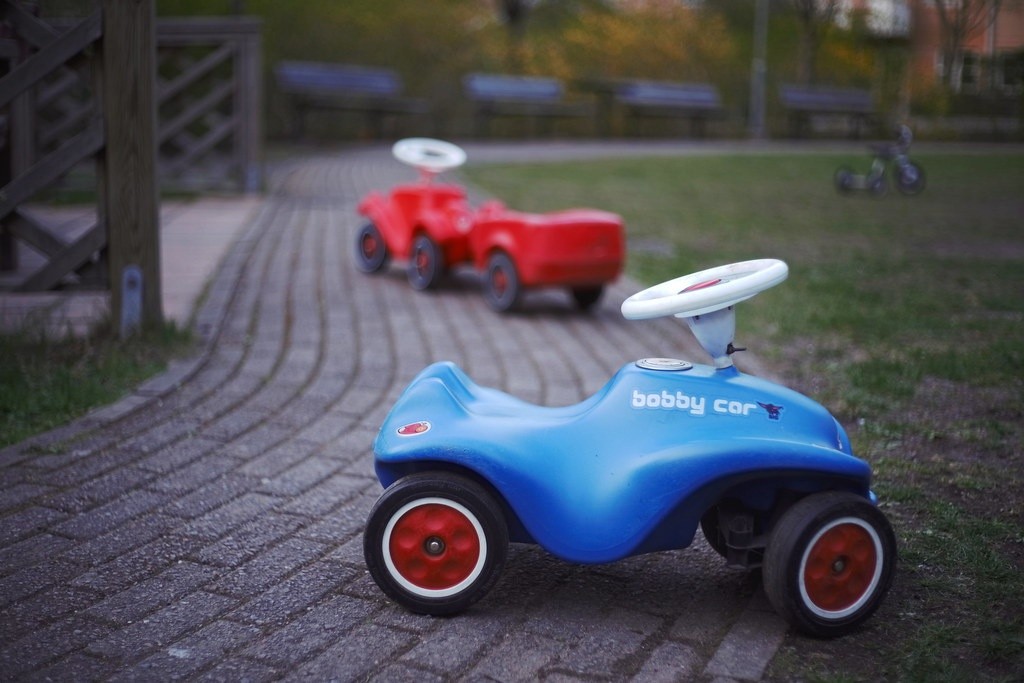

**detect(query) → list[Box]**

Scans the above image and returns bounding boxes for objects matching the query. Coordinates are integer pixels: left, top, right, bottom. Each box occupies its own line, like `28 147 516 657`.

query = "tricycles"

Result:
832 125 927 200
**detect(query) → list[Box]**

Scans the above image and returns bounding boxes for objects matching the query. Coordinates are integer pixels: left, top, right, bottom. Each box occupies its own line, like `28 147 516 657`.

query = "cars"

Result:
356 136 625 312
361 261 896 640
